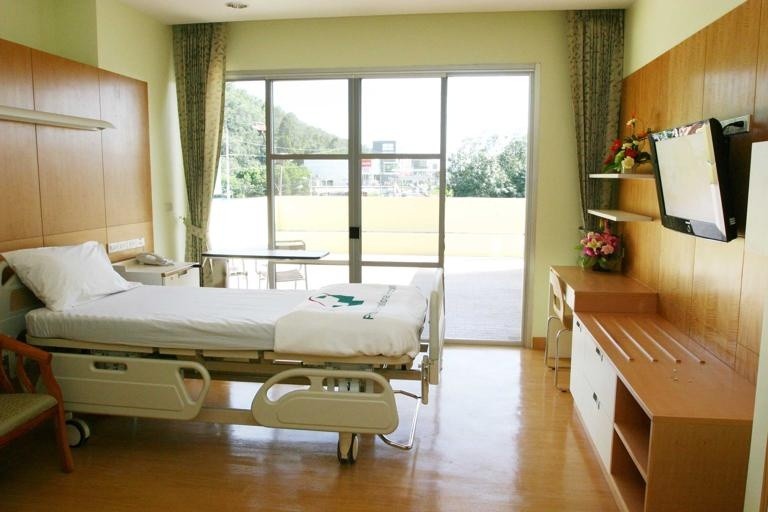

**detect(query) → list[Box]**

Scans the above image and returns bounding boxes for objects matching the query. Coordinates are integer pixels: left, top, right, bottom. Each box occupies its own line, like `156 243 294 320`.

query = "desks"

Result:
200 249 330 287
545 263 663 377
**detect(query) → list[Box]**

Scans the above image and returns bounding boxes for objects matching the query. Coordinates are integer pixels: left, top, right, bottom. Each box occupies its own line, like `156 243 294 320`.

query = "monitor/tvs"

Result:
646 118 739 243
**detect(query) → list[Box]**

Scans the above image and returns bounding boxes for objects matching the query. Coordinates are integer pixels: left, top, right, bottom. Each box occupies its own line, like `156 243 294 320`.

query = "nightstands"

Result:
110 254 204 287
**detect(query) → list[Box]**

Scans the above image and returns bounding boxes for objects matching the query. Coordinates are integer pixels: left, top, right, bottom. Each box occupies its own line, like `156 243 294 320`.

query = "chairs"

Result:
259 238 313 288
0 334 74 474
543 266 575 389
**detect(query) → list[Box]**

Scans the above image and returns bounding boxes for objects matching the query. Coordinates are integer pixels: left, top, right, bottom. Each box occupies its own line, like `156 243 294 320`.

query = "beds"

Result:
0 244 445 467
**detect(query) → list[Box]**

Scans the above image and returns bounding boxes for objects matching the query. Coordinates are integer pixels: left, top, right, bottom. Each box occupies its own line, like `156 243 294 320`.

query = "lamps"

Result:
0 103 117 132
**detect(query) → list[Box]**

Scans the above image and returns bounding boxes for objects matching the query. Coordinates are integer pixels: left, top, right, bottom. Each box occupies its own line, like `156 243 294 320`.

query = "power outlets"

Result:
734 114 749 134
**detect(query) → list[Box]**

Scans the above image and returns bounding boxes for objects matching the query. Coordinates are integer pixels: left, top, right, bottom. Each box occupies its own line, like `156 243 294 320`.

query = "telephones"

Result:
136 252 173 265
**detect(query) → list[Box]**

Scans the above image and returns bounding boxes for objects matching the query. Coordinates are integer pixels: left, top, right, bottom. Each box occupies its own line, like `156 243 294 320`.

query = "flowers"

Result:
575 223 626 272
601 114 653 173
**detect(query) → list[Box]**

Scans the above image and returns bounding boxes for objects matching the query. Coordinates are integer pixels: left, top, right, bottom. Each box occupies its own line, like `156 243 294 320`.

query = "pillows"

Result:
1 239 145 314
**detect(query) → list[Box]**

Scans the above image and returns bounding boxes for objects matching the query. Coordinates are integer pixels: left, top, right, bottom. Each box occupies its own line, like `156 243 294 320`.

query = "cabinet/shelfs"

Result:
568 305 757 511
584 172 657 226
